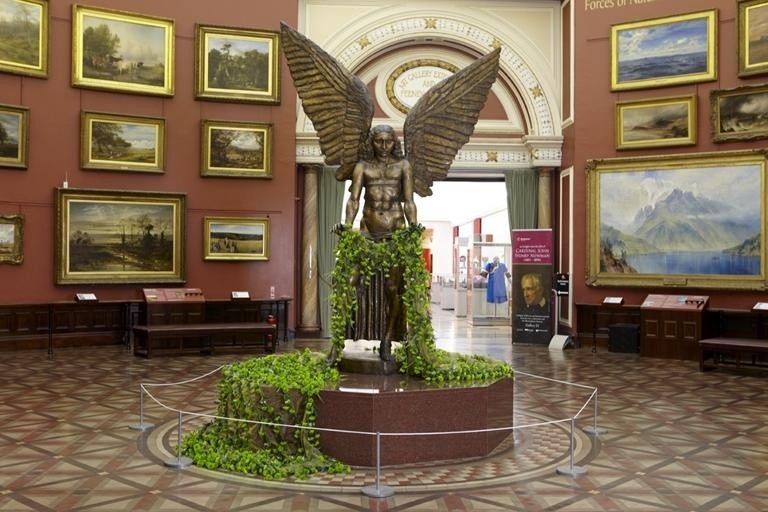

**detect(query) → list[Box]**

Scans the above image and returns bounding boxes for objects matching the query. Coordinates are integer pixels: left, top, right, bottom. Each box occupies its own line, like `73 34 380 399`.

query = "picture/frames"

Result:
0 103 31 169
709 84 768 144
0 0 48 79
200 119 273 180
0 215 25 266
610 8 717 92
194 22 281 106
80 110 165 175
585 148 768 292
71 3 176 98
614 93 697 151
55 187 187 286
736 0 768 79
203 215 270 261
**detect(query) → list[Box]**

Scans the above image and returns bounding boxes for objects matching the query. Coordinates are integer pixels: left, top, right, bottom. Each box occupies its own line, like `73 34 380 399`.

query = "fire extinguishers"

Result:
266 311 277 348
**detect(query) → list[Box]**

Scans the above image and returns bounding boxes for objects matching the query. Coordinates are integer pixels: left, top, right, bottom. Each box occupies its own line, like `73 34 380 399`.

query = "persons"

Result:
512 271 549 345
485 256 512 303
330 124 422 364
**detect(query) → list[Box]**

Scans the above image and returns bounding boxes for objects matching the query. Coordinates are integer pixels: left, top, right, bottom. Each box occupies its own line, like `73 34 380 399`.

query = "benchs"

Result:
130 322 275 359
698 336 768 372
